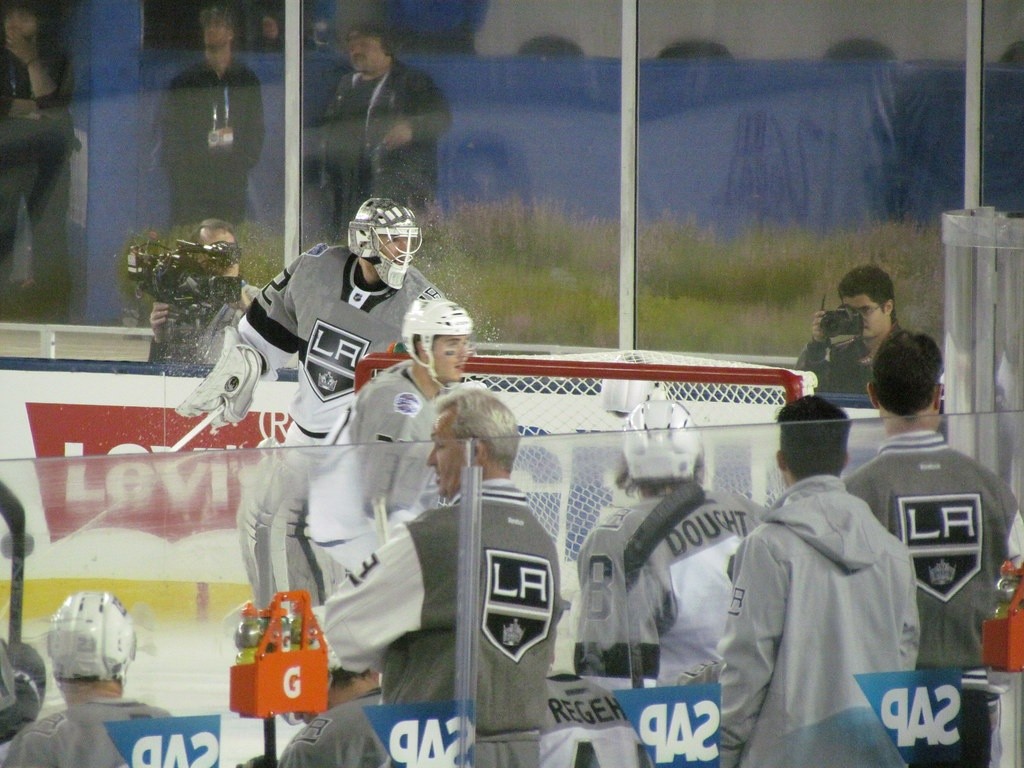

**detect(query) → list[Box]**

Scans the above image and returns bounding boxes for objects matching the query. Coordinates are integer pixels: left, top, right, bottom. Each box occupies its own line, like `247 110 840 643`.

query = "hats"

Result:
200 7 237 30
345 23 390 41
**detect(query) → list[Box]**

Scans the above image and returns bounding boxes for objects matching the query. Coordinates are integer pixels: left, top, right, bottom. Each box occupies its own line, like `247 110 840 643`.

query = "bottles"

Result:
236 600 313 664
995 559 1023 620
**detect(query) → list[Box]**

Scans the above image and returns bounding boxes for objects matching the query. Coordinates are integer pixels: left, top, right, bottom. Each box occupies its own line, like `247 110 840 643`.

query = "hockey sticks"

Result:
0 480 28 675
622 482 706 688
27 403 226 575
370 492 389 547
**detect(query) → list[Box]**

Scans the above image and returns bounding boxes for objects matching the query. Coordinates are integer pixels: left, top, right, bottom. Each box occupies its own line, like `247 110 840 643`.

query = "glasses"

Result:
852 297 889 317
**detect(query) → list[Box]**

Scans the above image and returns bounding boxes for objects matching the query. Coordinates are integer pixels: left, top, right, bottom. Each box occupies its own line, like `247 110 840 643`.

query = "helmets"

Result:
311 604 344 683
48 591 138 679
621 398 705 487
347 197 423 293
401 298 474 367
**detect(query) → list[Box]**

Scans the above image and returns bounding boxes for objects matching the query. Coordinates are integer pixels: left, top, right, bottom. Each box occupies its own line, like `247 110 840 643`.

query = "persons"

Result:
794 265 903 395
160 6 265 226
537 671 656 768
316 28 449 241
308 295 475 573
841 327 1024 767
146 219 264 367
278 606 389 768
322 390 561 768
0 591 174 768
715 396 921 768
0 0 78 324
573 400 765 693
175 195 452 607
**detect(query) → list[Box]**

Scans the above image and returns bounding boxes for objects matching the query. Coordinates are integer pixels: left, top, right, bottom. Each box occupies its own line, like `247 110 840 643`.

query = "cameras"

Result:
819 304 864 337
